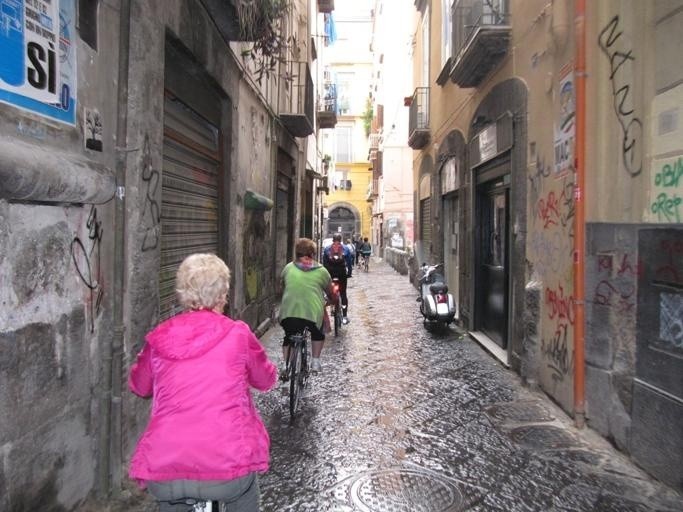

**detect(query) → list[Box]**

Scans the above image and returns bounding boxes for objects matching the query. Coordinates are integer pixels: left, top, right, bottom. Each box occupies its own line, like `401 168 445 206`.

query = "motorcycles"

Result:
416 262 456 334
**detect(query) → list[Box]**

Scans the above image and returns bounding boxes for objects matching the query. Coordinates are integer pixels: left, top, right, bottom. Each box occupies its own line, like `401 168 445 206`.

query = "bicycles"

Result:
357 250 370 273
327 273 351 337
279 296 338 419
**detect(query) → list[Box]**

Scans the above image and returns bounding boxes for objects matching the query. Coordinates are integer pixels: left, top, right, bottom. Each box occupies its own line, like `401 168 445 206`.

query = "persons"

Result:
321 232 352 325
351 236 359 264
357 237 362 244
359 237 371 267
321 233 333 249
276 237 339 382
127 250 278 512
345 238 355 266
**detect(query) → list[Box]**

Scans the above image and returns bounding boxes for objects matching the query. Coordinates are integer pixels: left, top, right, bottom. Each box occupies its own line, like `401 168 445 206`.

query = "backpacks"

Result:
328 243 344 263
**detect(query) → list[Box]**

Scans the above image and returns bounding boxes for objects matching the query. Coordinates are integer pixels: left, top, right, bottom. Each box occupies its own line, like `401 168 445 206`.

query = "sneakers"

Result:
342 317 351 325
278 358 322 382
